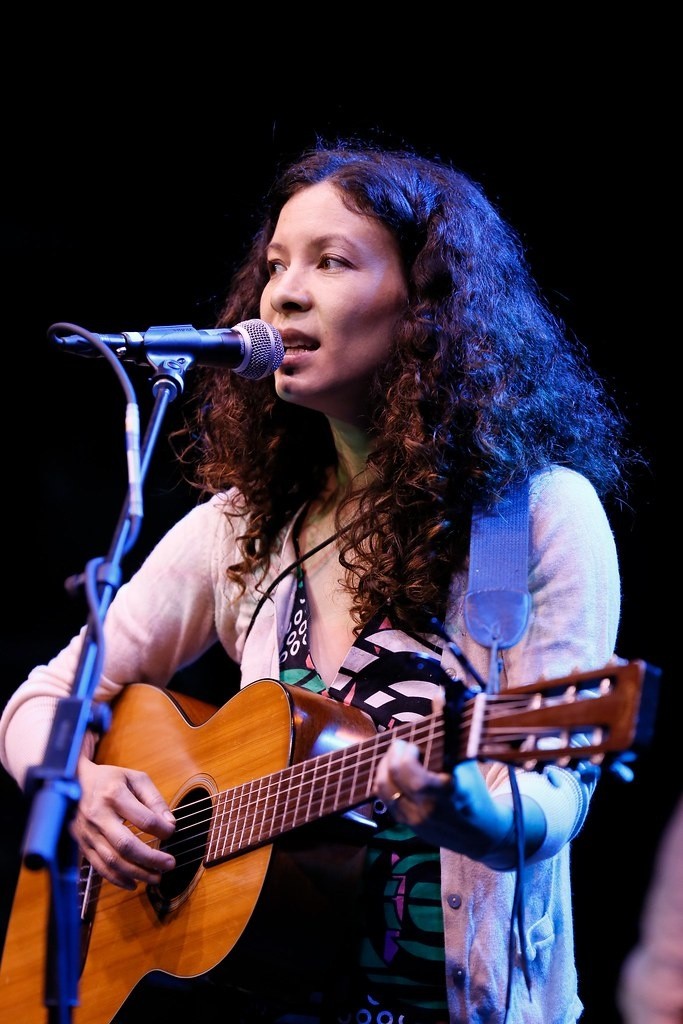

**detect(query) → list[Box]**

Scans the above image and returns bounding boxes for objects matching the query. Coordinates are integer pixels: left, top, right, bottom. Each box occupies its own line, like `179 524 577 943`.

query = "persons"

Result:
0 145 623 1023
619 801 683 1024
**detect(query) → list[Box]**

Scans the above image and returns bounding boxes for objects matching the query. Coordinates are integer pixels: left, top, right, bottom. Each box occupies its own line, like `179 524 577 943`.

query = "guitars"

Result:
0 656 662 1024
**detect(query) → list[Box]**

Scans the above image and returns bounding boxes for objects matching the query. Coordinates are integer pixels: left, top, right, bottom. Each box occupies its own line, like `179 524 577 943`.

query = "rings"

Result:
391 791 400 801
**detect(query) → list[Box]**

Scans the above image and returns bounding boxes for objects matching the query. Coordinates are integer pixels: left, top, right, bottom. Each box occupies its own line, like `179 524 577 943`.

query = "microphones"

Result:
59 319 285 380
